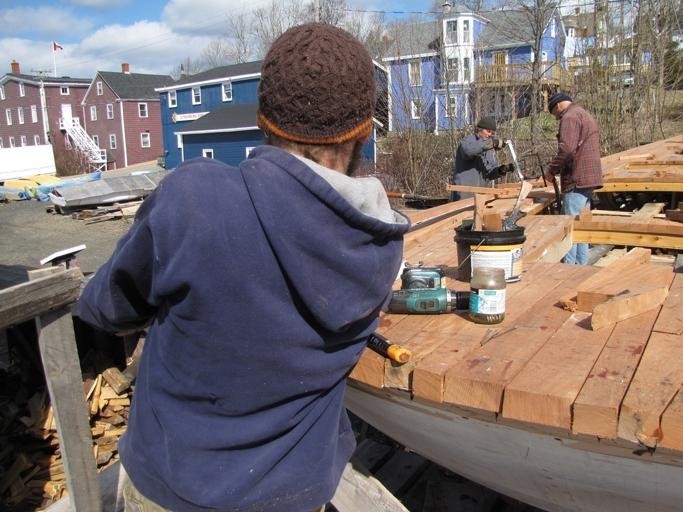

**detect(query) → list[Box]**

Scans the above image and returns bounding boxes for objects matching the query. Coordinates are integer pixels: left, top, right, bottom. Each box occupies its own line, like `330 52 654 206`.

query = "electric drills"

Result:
390 268 469 314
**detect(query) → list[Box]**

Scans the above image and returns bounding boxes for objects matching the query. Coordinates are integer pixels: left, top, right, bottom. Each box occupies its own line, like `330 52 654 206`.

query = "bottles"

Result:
468 265 507 324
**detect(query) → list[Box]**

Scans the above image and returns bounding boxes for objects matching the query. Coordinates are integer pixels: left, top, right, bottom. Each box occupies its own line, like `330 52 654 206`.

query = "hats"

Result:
476 116 497 130
257 21 377 144
547 92 572 114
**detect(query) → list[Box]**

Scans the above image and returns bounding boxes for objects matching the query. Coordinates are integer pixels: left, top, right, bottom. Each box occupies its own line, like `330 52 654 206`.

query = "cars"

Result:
609 69 640 92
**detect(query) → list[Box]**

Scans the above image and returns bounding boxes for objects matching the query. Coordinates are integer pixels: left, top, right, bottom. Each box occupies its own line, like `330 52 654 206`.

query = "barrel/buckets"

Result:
453 223 527 283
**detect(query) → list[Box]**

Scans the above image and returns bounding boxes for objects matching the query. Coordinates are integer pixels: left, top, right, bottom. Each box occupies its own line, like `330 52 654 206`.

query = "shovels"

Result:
508 181 535 223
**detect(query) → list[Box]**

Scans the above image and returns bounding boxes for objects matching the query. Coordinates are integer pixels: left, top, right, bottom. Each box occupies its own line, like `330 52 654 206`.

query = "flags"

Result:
51 40 64 53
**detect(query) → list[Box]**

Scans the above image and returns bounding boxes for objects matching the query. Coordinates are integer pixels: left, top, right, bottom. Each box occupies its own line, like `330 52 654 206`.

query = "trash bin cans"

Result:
157 157 163 165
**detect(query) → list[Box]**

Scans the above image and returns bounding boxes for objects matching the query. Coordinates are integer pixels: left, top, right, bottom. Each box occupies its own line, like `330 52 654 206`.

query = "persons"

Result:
543 94 604 215
453 116 516 200
75 22 411 510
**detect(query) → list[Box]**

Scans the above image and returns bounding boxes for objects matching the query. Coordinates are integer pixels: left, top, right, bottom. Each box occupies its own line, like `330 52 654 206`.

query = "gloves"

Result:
504 162 515 172
491 139 505 150
